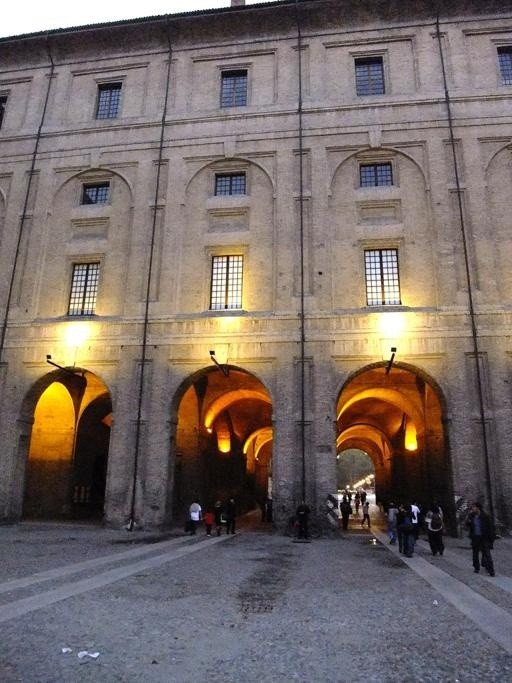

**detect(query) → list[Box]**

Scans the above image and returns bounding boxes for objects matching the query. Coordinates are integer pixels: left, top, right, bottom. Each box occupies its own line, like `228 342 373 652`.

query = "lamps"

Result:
385 347 396 375
45 354 84 379
209 350 229 377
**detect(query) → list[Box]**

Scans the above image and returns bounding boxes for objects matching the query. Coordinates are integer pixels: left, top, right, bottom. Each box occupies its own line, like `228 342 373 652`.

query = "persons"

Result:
225 498 239 535
467 501 497 576
295 499 311 538
424 499 445 556
202 506 215 536
339 487 373 530
384 498 422 557
188 497 203 535
213 498 225 536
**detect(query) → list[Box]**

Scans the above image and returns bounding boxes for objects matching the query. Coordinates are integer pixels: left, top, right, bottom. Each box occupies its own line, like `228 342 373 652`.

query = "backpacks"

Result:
430 510 443 529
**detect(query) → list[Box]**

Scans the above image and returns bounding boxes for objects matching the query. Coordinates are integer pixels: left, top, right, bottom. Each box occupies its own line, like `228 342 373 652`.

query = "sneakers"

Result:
472 559 495 576
389 538 397 545
432 549 444 556
399 549 415 558
190 530 236 537
